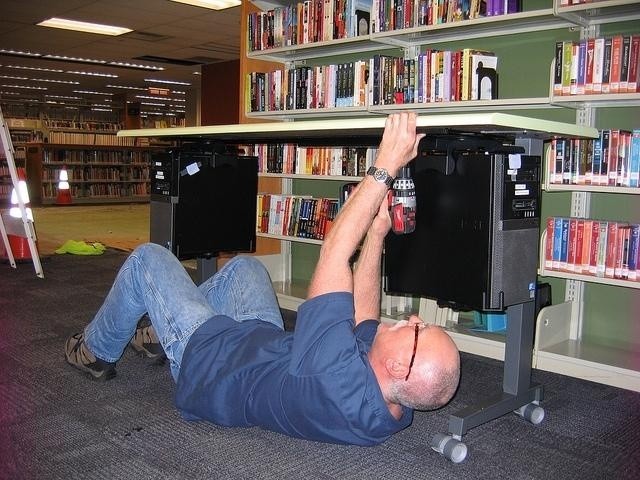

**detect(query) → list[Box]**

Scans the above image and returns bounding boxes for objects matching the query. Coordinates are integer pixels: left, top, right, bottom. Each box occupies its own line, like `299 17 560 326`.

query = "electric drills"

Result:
339 163 417 235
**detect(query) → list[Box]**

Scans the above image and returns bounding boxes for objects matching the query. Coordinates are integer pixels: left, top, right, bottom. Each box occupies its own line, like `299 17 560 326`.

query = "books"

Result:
0 117 186 199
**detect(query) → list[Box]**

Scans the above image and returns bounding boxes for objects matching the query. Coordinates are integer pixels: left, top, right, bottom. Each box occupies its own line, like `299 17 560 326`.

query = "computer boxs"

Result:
385 151 542 311
150 151 258 259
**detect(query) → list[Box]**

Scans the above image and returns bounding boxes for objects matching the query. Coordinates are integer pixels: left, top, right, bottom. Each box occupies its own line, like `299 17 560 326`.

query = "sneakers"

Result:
130 315 167 362
64 330 117 381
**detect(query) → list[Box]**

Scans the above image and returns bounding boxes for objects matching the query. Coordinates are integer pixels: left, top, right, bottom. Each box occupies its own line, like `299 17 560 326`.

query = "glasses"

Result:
405 322 427 382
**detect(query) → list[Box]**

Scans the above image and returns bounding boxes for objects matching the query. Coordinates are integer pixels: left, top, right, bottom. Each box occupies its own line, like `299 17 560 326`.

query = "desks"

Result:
116 111 600 465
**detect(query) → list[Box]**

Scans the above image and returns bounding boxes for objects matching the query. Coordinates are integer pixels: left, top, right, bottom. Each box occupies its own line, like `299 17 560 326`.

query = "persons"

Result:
65 111 461 448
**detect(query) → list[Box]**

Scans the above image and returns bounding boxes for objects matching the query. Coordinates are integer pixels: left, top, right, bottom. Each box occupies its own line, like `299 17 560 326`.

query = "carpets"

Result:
0 244 640 478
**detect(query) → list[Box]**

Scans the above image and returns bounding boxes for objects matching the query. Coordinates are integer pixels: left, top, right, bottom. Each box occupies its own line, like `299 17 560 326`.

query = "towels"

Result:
54 239 106 256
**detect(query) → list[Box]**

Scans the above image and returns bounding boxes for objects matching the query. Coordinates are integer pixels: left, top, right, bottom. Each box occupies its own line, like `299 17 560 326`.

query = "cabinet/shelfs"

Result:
0 116 185 206
233 0 640 391
